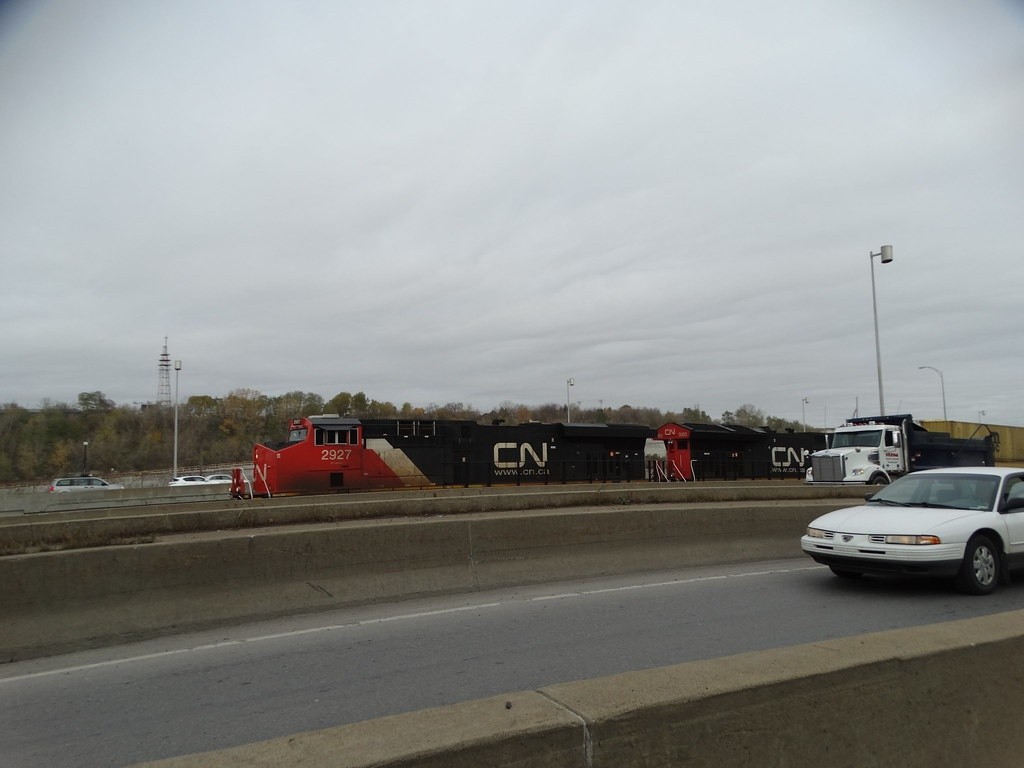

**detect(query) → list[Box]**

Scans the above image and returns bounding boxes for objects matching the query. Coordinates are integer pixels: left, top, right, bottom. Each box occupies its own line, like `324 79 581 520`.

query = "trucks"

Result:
803 414 1000 485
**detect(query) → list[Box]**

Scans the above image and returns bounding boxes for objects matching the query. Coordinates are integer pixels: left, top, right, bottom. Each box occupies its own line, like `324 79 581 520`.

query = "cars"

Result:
800 467 1024 596
206 475 248 484
50 476 124 493
168 476 212 486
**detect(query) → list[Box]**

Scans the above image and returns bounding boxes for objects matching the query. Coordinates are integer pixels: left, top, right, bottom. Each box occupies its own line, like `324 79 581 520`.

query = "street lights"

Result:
871 245 893 416
802 397 810 431
174 360 182 478
918 366 947 420
566 378 574 424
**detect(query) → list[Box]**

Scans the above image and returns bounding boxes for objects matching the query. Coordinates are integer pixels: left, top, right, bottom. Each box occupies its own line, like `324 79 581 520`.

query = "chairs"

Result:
936 490 961 503
975 486 1005 505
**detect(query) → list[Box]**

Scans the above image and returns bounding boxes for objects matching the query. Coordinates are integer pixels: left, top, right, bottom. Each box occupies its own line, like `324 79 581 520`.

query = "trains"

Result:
255 412 834 498
